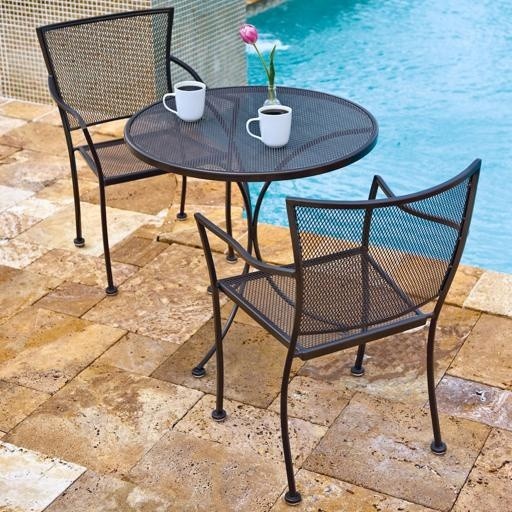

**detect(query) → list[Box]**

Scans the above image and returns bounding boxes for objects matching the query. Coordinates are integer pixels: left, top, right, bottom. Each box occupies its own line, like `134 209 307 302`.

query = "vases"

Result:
260 86 282 108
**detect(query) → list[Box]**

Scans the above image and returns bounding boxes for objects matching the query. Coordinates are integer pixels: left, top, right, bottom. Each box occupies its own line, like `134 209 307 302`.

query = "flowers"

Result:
238 21 281 87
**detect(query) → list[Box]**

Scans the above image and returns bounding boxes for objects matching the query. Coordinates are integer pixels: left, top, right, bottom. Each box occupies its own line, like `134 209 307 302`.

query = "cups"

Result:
162 80 206 125
245 104 292 149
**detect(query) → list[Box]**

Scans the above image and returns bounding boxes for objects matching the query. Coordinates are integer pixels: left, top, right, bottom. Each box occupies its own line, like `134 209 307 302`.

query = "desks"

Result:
123 84 378 378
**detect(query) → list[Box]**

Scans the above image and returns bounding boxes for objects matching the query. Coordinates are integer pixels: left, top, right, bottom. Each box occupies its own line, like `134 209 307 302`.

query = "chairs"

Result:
193 157 485 504
36 8 237 297
